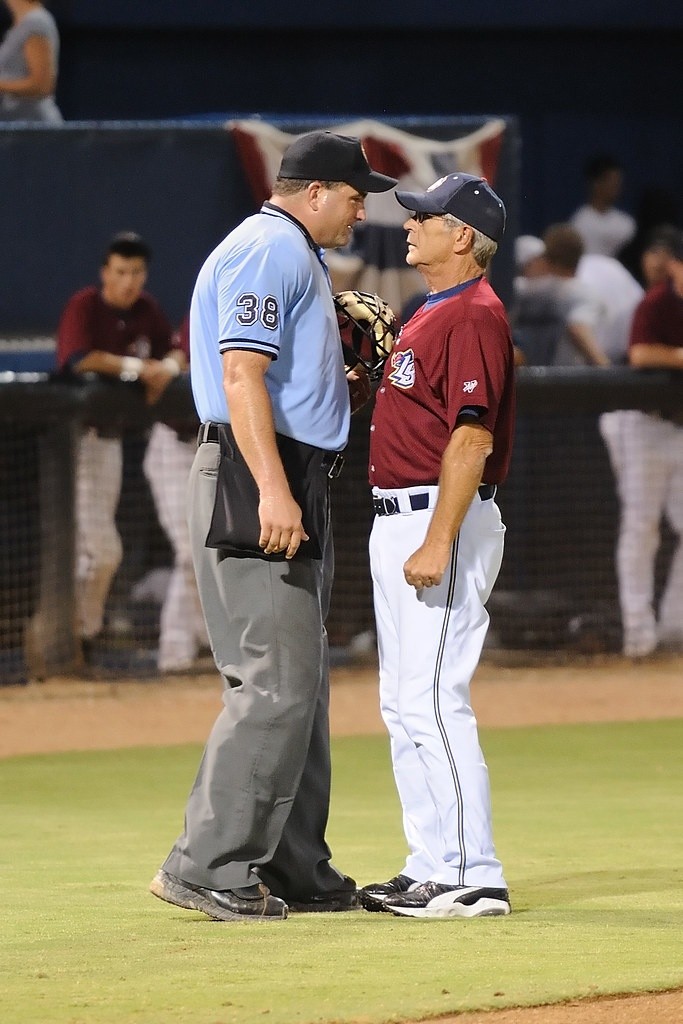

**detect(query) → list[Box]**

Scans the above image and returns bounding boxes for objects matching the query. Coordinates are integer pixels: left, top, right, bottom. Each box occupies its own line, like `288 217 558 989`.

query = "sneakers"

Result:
151 866 289 921
382 880 511 919
360 875 416 911
270 875 361 913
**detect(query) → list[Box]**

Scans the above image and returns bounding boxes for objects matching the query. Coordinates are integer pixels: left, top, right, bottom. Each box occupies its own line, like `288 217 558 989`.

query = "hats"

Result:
545 226 583 271
279 131 398 193
396 173 506 243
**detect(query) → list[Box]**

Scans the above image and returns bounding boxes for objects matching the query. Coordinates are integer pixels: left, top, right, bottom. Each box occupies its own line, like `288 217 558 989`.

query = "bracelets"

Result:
158 359 181 378
123 354 142 380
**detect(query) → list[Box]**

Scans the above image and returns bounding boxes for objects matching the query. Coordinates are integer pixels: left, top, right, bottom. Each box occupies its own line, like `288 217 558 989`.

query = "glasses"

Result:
417 212 462 223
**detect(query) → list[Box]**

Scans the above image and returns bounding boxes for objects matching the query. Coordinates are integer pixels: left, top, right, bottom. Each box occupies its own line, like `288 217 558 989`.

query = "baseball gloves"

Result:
333 289 398 380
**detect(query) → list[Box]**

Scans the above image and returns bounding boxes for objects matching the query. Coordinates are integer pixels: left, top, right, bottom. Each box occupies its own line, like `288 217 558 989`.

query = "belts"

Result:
372 482 494 517
199 424 344 479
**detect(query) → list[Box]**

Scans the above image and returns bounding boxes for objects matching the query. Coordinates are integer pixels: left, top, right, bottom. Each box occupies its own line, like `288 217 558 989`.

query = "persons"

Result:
598 240 683 658
21 230 173 683
510 156 644 369
357 175 516 917
143 294 399 672
0 0 62 124
146 128 402 923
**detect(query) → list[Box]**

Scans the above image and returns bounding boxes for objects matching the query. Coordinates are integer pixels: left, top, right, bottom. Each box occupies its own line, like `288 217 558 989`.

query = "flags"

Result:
232 124 510 368
